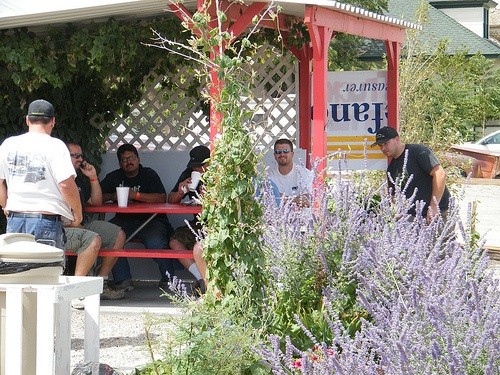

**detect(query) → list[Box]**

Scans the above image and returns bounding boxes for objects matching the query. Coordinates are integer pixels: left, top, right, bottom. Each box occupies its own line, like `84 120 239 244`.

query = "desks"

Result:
84 202 202 278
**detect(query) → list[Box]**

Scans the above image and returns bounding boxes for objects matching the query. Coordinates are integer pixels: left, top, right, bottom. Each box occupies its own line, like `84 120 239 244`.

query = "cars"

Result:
420 130 500 179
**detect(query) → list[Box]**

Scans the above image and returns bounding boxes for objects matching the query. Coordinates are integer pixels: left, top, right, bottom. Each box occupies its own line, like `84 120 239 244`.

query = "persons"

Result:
370 126 453 259
98 143 173 294
168 146 214 298
0 100 84 275
60 141 127 299
259 139 312 234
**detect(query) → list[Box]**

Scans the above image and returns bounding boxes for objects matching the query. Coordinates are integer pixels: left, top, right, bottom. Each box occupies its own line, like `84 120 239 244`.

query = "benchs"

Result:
64 247 195 258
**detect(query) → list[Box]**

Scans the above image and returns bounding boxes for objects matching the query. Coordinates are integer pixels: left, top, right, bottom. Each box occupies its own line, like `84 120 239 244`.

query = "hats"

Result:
370 127 398 148
186 145 210 169
27 99 54 118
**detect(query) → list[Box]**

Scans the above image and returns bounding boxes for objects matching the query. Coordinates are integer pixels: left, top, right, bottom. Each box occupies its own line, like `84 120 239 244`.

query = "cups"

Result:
116 187 129 207
189 170 202 192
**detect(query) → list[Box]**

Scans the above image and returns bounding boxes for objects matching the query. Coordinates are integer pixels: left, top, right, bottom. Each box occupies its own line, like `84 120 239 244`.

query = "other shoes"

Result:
71 297 85 309
100 284 127 300
159 278 173 293
112 279 135 292
198 279 207 295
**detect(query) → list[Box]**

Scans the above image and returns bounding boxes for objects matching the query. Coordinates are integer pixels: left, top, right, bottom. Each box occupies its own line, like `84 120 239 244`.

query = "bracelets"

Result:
89 177 98 182
135 191 141 201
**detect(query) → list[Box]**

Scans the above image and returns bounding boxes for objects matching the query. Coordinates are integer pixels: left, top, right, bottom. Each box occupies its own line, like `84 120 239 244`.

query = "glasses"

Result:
71 153 85 159
121 155 137 161
274 149 291 154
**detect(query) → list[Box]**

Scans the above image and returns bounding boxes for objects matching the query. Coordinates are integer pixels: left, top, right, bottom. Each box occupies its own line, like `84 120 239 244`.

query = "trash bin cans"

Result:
0 232 65 375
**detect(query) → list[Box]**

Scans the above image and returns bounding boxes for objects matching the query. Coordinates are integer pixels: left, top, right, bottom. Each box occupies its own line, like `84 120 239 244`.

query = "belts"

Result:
8 211 62 222
